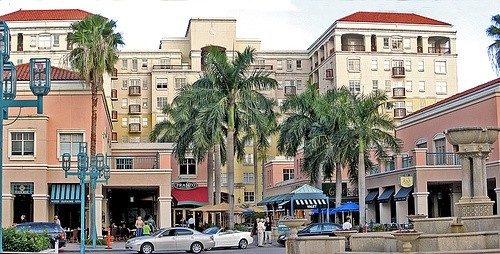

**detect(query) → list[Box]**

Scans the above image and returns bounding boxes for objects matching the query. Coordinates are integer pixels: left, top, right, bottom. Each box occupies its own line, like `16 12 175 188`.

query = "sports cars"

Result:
201 226 254 249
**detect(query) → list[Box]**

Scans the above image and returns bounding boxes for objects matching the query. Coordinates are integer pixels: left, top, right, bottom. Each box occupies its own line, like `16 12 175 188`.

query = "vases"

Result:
391 231 423 252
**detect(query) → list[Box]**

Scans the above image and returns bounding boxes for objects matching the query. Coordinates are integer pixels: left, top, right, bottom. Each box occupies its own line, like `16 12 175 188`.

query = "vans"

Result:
384 222 414 231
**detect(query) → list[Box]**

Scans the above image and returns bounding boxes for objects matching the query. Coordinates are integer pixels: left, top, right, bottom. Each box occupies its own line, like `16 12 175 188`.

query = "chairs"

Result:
110 224 129 241
68 229 82 243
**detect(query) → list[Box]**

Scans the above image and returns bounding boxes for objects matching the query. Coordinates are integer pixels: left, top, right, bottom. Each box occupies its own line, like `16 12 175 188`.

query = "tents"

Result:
311 202 368 224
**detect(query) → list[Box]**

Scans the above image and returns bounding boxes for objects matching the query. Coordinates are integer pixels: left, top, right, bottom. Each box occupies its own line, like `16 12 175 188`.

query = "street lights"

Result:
61 142 110 254
0 19 52 252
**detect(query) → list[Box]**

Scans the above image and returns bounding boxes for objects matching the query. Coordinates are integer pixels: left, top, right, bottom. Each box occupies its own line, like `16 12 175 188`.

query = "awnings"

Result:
377 188 394 203
365 191 378 204
394 187 413 201
171 187 208 207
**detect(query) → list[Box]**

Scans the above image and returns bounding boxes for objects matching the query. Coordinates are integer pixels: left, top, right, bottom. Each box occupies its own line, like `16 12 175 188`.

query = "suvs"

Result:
14 222 66 248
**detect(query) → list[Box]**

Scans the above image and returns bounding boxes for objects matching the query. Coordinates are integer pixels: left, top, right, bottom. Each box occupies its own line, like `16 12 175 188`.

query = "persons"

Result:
134 216 155 236
199 220 202 232
252 217 272 247
187 217 194 229
180 216 186 228
53 215 60 225
20 215 27 223
342 218 352 230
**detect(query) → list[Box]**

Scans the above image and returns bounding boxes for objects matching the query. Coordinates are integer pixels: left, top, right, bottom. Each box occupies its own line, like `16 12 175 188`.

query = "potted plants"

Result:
234 225 251 237
373 224 385 232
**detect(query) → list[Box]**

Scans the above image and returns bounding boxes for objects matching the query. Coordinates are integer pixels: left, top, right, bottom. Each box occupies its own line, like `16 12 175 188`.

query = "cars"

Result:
351 225 364 233
125 227 215 254
277 223 344 247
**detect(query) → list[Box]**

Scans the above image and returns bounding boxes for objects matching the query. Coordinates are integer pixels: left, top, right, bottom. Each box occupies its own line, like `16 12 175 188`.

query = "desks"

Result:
64 229 87 242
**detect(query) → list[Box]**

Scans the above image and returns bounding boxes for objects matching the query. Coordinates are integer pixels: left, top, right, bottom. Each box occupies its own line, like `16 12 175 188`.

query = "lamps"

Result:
238 197 241 203
